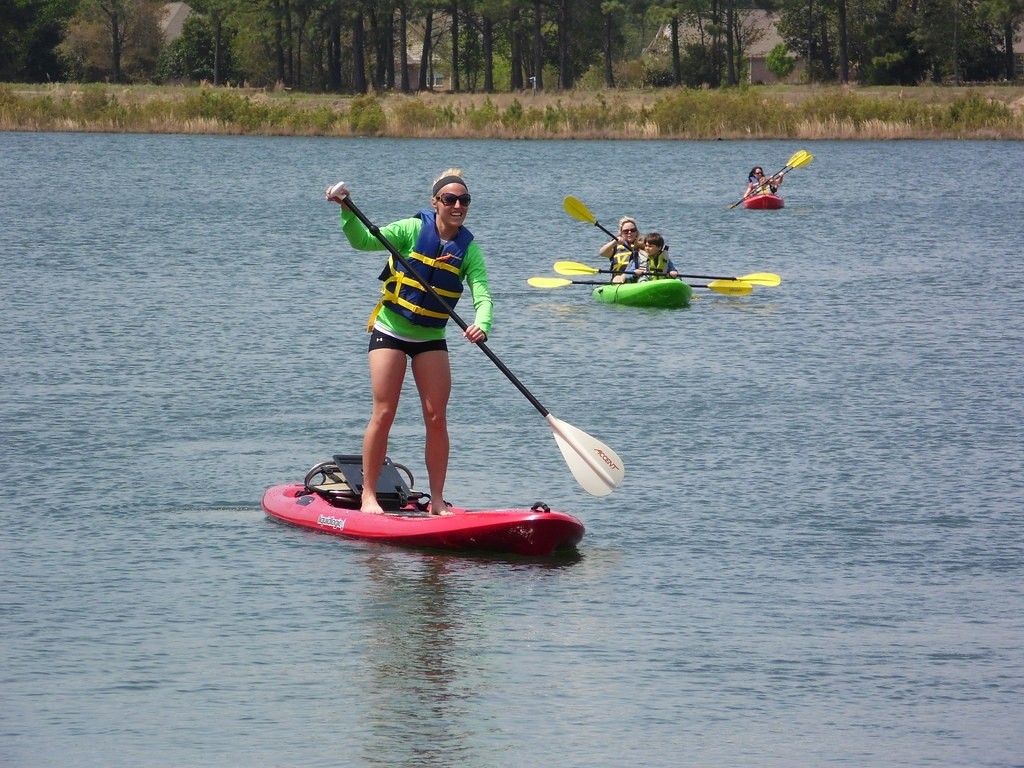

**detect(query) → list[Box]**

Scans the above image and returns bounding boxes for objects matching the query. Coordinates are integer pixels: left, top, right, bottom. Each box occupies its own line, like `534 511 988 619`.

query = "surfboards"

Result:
260 455 585 558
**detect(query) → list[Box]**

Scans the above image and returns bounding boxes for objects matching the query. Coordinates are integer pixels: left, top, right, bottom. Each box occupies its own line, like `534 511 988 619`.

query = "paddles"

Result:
730 149 812 210
325 180 624 499
525 195 782 297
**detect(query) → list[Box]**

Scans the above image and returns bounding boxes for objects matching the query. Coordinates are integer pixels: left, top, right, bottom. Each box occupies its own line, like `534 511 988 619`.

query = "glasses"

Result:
436 193 471 206
622 228 636 234
756 172 763 174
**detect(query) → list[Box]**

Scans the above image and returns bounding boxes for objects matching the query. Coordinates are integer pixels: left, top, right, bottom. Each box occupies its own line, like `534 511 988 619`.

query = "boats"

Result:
589 278 694 311
261 454 588 560
742 194 784 209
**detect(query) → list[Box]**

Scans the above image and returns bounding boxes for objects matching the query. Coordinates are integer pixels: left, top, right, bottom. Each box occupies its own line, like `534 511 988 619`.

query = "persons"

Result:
625 233 678 284
743 166 784 200
753 176 778 197
325 168 493 516
600 216 645 285
